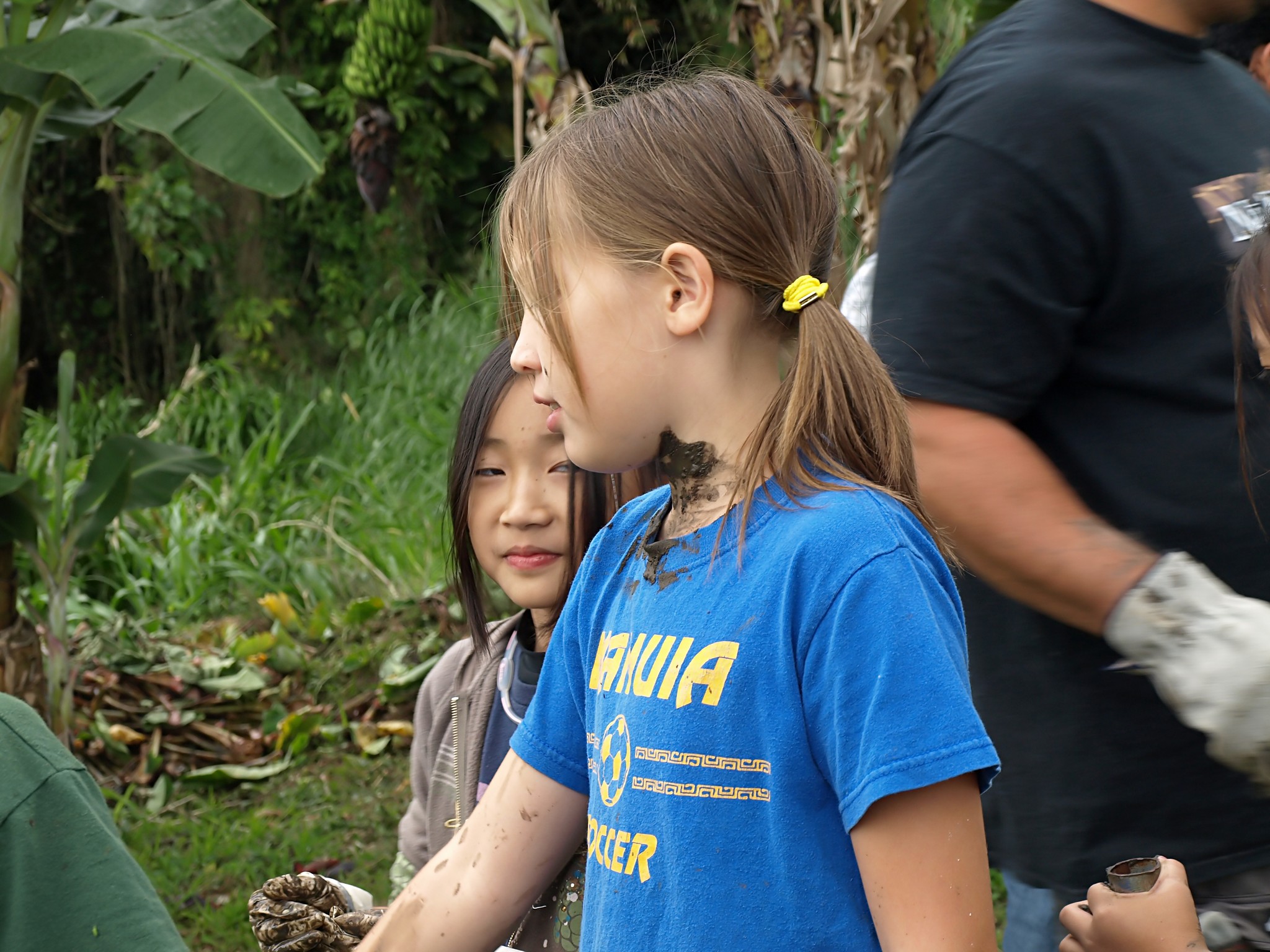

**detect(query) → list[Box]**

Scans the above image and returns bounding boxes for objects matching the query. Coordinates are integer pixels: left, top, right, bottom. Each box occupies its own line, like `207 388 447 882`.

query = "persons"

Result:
0 689 191 952
245 0 1270 952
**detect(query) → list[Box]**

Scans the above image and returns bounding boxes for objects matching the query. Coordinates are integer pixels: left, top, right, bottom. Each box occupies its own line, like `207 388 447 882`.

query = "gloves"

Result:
248 868 388 952
1105 552 1270 783
